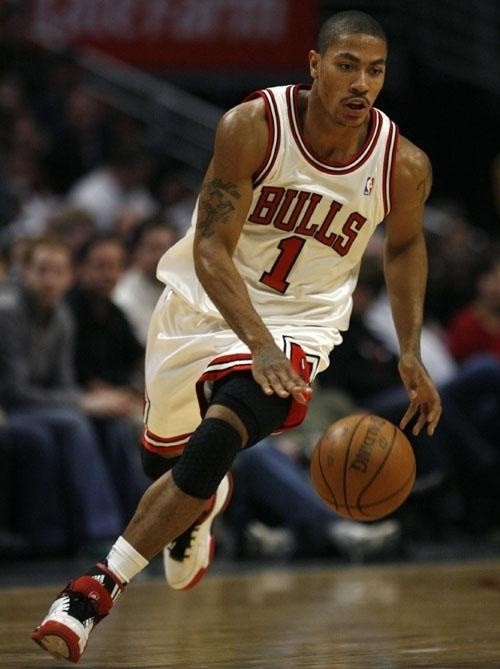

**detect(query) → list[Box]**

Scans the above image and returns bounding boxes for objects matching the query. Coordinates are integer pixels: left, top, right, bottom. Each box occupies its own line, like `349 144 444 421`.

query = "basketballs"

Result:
310 415 416 521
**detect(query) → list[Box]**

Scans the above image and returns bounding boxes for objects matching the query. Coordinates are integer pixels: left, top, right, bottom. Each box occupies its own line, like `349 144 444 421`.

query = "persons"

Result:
1 3 500 575
28 8 444 664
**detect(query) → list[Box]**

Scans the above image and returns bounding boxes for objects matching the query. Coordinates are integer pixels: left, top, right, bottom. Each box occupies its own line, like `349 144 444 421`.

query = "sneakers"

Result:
31 558 128 662
164 471 233 592
